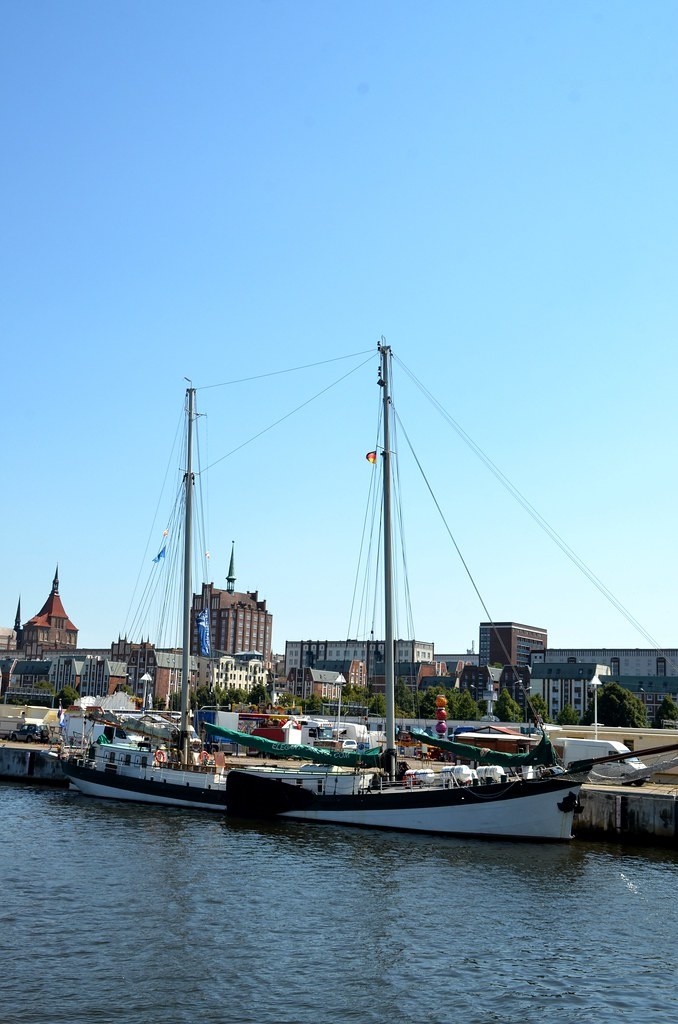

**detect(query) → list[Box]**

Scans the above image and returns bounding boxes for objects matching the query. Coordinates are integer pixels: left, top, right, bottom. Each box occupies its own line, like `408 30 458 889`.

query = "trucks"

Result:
246 720 333 758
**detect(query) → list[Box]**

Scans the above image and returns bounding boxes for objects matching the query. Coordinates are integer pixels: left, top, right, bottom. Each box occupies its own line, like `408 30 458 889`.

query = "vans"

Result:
93 724 145 744
555 738 650 786
163 723 201 744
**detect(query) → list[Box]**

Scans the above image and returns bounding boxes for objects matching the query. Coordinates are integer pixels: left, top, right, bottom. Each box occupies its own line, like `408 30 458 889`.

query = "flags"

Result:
152 546 166 564
196 609 211 657
366 451 378 463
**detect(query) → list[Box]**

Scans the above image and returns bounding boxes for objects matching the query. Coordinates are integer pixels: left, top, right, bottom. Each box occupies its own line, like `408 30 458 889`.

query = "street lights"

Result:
525 686 532 723
492 674 494 721
513 680 522 723
167 660 170 694
640 688 646 728
471 685 477 721
172 659 175 711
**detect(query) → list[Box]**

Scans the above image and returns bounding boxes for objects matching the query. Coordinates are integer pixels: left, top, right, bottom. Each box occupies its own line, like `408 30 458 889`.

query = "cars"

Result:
342 740 358 751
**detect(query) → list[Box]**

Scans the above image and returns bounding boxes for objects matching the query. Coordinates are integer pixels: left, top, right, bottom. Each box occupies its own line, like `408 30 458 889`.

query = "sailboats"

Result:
59 334 586 845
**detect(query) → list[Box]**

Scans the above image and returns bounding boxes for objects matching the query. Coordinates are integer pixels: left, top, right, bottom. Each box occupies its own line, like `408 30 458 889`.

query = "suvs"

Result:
11 724 50 743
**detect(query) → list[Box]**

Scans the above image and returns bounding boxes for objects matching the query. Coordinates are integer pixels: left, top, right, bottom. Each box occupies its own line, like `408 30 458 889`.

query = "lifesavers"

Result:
155 750 165 763
200 751 209 762
211 748 216 754
402 773 415 788
192 740 201 752
